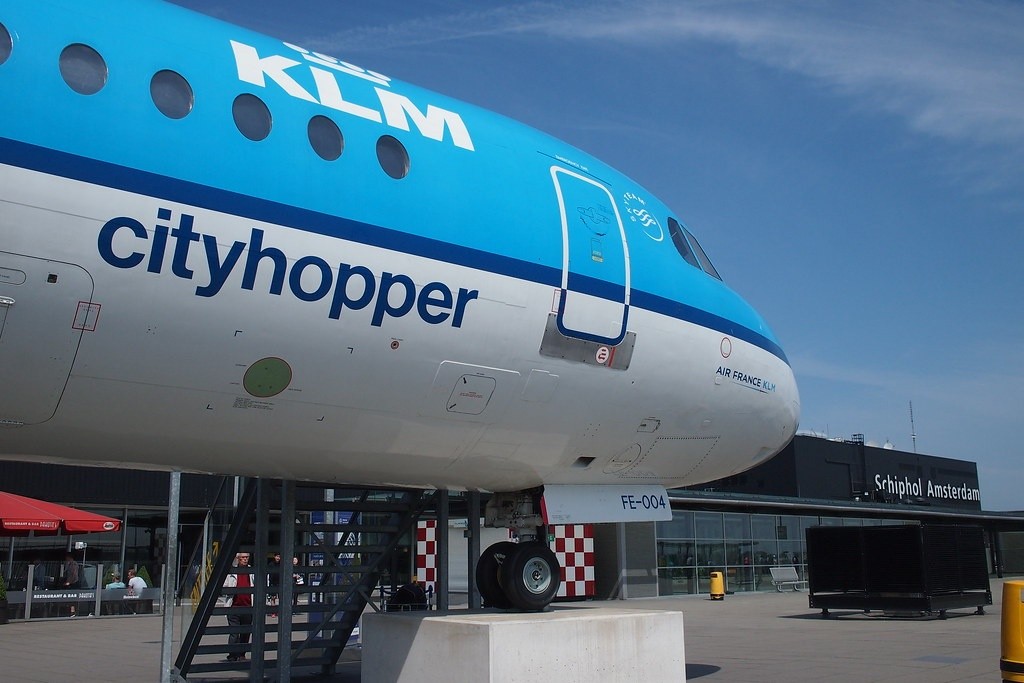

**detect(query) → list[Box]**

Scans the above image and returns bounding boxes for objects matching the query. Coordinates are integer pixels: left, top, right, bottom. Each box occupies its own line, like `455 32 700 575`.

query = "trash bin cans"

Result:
999 579 1024 683
710 571 726 601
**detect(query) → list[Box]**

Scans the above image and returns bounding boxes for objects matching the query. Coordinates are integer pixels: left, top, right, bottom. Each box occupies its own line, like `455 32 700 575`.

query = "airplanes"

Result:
0 0 801 612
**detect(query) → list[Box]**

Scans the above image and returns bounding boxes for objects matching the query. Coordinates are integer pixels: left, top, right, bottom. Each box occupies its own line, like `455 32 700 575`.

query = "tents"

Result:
0 490 124 588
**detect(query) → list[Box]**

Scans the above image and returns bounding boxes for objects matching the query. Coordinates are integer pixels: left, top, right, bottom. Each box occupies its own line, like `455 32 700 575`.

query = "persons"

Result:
666 549 808 583
221 554 300 661
32 553 148 617
